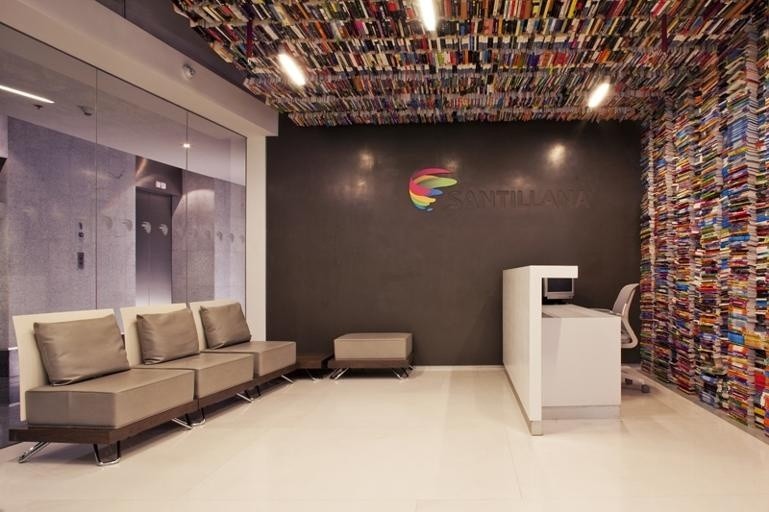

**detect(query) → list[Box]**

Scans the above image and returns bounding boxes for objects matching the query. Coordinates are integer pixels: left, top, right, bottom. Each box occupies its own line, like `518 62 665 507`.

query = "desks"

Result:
541 302 621 426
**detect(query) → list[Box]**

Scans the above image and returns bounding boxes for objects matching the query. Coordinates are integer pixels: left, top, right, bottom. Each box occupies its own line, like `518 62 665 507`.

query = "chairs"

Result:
592 283 650 395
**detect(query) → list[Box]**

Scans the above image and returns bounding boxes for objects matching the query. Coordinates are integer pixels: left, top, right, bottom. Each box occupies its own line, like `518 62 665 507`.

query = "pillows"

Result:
32 303 252 386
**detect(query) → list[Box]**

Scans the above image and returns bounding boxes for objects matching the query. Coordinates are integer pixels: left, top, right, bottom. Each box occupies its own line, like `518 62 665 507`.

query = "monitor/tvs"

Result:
544 279 575 300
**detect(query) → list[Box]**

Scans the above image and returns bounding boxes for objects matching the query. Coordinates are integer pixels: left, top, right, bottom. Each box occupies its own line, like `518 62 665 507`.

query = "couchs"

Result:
8 301 297 468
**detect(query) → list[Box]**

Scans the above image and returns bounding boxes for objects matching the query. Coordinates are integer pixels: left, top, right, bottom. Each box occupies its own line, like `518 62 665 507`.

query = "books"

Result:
173 1 767 128
639 128 769 443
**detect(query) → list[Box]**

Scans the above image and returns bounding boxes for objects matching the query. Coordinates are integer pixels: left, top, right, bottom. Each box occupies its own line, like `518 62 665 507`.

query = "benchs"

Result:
298 332 417 380
